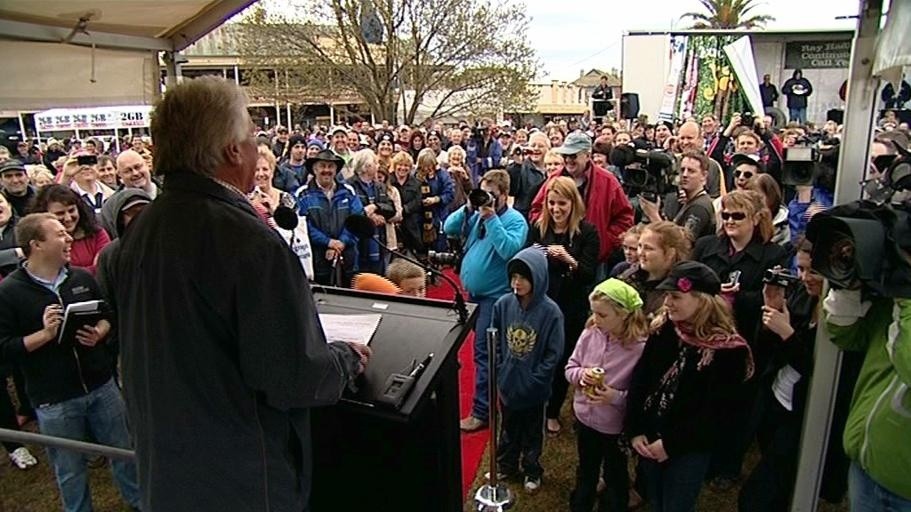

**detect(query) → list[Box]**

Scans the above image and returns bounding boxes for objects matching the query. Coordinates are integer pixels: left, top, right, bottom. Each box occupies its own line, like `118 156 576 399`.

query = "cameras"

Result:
467 187 499 213
77 155 98 165
761 267 799 289
742 111 754 127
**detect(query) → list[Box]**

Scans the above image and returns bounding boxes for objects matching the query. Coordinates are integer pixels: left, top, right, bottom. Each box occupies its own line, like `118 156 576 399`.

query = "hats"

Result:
47 135 59 147
503 118 678 139
302 149 345 176
730 152 763 171
553 132 594 157
119 195 154 211
654 260 724 295
1 158 26 173
277 124 445 151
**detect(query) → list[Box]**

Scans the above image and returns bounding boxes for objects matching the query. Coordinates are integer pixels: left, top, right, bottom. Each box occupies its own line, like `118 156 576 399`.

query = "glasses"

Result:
721 209 752 221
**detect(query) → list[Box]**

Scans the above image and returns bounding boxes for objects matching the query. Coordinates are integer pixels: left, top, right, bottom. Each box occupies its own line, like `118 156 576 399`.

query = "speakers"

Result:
621 93 639 117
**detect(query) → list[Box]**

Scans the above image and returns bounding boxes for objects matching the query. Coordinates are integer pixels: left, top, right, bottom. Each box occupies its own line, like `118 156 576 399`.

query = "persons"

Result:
0 213 141 509
841 72 909 109
625 258 753 510
443 170 529 432
528 176 600 433
353 273 402 295
110 73 371 508
490 248 565 495
527 130 635 278
253 121 402 288
565 109 909 510
565 278 650 511
389 119 551 280
760 74 779 107
2 136 164 471
782 71 812 124
385 258 424 298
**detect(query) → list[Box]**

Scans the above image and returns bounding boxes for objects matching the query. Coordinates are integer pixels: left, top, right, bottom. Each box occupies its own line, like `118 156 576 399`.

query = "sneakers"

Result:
592 471 643 507
7 445 38 469
14 404 36 426
521 467 542 492
484 467 519 481
459 414 490 432
546 412 561 437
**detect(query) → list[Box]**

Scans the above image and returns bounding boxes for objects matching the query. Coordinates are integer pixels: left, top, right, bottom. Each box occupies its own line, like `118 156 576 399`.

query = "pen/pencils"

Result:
409 352 435 378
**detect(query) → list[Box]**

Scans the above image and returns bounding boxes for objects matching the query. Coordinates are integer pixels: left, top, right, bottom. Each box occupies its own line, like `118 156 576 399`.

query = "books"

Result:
60 310 102 347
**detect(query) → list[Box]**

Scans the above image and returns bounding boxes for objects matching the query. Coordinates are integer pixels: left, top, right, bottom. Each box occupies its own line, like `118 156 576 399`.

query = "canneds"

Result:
581 366 604 398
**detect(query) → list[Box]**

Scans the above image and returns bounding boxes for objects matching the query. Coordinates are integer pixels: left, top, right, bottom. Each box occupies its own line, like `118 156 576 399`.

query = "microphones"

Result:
270 205 300 251
341 209 469 322
